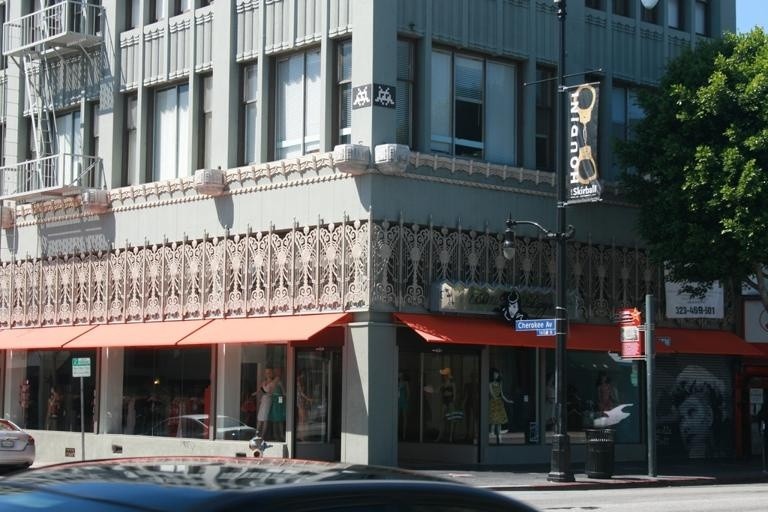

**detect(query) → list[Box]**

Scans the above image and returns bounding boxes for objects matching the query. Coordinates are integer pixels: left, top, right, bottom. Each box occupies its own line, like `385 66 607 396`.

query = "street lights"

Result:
502 213 578 484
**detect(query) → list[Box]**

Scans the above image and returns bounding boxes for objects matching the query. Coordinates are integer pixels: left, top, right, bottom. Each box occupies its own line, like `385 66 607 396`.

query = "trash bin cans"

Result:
582 428 616 479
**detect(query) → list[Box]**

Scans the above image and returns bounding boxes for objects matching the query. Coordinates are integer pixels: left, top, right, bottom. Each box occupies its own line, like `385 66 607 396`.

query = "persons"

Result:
249 367 273 439
596 371 624 418
44 384 64 428
296 367 317 442
268 368 286 442
398 370 411 440
489 368 516 444
673 378 728 461
429 368 456 443
746 397 768 472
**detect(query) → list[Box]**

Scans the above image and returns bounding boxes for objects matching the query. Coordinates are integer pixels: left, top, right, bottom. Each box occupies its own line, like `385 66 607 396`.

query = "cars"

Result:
0 418 35 470
0 457 543 510
585 402 635 442
143 412 256 441
